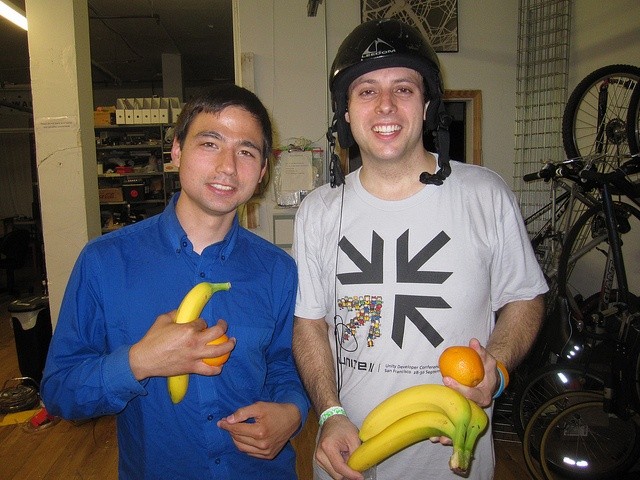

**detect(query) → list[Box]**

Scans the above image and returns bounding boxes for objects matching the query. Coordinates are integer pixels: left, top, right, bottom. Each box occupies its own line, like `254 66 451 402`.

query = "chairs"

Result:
0 229 34 301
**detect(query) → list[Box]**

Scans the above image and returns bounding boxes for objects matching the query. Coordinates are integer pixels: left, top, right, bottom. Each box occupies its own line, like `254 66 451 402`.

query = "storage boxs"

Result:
123 184 145 201
269 147 324 209
98 185 123 202
93 105 116 126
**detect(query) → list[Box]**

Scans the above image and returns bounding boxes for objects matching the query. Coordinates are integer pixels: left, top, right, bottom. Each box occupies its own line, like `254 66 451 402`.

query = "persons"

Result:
40 86 313 480
292 20 548 480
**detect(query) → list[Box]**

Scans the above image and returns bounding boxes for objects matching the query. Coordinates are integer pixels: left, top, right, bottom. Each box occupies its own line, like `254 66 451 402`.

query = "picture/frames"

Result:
360 1 459 54
333 90 483 178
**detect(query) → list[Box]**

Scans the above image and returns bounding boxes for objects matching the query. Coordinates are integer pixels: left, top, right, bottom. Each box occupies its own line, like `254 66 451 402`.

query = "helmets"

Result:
328 18 444 149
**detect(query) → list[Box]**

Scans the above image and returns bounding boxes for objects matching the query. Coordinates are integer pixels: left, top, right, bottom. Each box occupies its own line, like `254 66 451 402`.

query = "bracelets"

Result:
497 361 510 389
493 368 504 399
319 406 348 426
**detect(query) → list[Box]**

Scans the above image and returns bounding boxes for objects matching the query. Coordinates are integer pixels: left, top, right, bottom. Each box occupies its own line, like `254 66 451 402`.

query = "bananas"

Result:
348 383 489 477
167 281 231 406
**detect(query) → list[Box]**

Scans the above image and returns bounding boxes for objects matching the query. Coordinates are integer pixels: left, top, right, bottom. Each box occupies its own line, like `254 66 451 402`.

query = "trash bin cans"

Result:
8 296 54 394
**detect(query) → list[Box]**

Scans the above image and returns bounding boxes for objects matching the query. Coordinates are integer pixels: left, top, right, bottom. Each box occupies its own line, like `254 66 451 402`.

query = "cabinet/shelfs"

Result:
93 125 183 236
